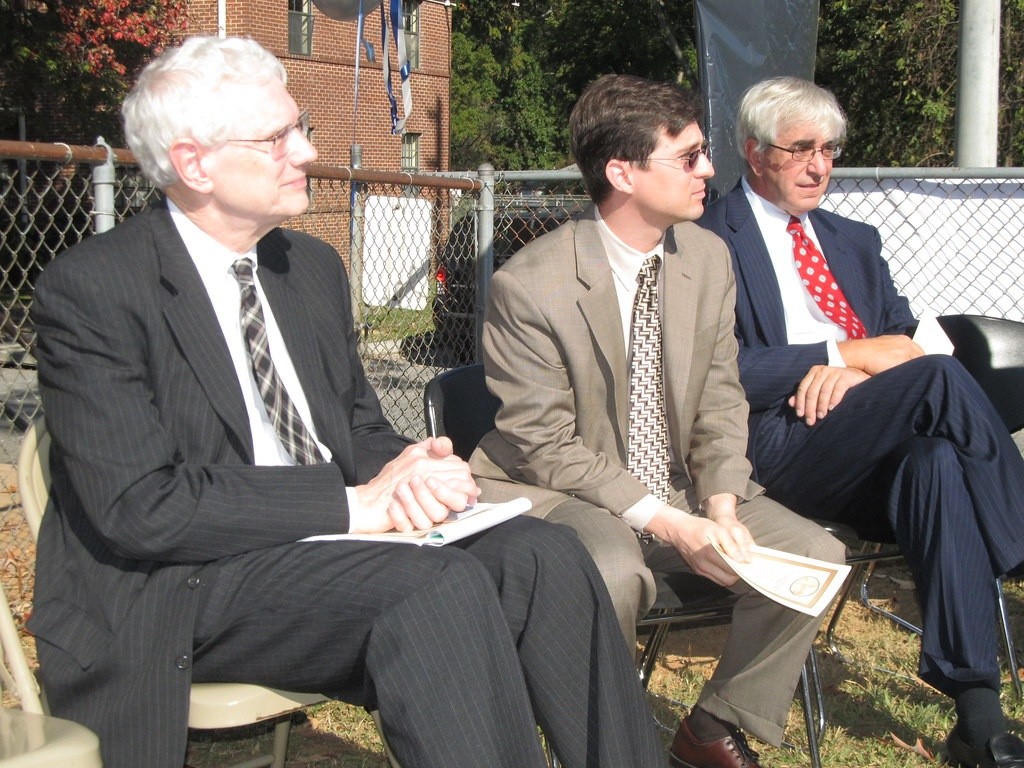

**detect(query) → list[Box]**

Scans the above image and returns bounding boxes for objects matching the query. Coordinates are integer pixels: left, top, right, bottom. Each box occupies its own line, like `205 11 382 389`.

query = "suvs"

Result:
433 206 593 362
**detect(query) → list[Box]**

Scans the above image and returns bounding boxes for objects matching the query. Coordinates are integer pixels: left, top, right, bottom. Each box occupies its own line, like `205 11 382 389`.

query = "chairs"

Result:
0 579 104 767
423 364 822 768
21 400 344 768
859 315 1023 639
815 518 1023 705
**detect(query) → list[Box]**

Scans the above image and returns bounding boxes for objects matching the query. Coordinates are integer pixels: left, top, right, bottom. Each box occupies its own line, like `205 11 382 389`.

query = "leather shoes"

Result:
946 722 1024 768
668 714 763 768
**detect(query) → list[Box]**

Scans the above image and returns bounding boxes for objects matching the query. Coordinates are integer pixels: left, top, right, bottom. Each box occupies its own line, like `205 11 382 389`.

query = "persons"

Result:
695 75 1024 767
463 69 852 767
23 34 666 768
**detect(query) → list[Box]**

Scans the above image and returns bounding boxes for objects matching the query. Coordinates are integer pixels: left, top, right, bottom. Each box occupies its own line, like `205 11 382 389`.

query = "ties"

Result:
227 256 328 466
625 255 672 546
786 215 869 340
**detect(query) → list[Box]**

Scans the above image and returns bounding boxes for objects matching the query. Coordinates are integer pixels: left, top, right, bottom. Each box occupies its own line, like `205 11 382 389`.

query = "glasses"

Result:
226 106 311 148
765 142 845 162
619 139 712 168
